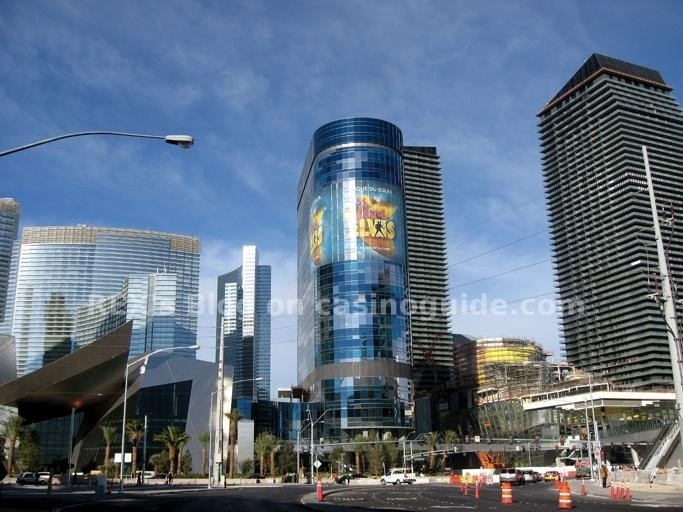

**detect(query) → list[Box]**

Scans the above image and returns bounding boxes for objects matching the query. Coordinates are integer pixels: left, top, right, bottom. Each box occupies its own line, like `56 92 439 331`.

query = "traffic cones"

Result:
579 478 587 496
552 473 563 489
608 478 632 503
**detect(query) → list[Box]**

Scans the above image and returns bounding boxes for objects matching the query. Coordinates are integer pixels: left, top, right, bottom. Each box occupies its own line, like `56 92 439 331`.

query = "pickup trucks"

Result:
380 467 416 485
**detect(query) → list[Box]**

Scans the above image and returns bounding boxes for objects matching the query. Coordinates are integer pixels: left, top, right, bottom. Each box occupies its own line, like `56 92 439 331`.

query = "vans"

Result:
500 469 524 484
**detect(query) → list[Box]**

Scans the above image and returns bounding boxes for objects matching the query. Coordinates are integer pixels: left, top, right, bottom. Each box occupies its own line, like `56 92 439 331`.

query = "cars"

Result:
520 465 634 482
334 473 367 485
16 469 84 485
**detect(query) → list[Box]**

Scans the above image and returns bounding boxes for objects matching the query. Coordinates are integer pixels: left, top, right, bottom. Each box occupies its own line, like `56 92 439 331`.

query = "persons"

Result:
600 465 608 488
168 472 173 485
374 220 386 238
136 471 142 485
165 476 169 485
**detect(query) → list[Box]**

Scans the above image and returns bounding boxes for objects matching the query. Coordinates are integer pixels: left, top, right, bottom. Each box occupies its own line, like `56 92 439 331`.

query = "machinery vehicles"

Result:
474 448 506 469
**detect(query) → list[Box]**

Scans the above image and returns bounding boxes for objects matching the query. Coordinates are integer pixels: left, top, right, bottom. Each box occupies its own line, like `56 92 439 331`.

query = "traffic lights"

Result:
508 433 513 445
560 435 563 445
535 436 539 452
464 435 469 444
486 435 491 443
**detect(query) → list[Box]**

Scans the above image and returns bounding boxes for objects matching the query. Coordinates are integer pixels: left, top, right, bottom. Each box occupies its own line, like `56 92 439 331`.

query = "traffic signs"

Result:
474 435 480 442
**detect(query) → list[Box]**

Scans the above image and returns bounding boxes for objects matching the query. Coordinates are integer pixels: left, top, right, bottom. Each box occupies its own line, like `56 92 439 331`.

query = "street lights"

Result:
0 130 196 154
296 420 325 474
207 377 264 487
305 405 347 482
564 371 602 487
118 344 200 487
401 429 432 474
